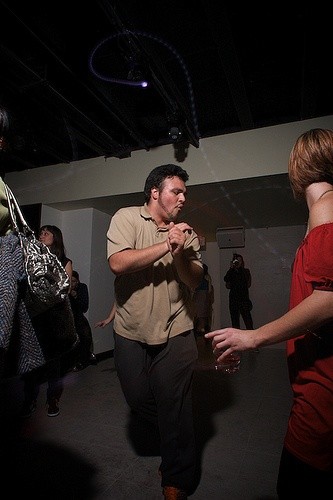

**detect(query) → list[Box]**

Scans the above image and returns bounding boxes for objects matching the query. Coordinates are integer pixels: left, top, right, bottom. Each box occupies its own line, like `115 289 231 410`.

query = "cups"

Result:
214 343 240 374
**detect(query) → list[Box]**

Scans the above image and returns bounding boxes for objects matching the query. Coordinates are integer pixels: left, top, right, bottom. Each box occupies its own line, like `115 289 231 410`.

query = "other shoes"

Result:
20 400 39 418
46 397 61 417
162 485 189 500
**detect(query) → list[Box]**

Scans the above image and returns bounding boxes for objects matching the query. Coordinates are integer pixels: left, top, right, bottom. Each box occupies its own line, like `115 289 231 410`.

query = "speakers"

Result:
216 227 246 248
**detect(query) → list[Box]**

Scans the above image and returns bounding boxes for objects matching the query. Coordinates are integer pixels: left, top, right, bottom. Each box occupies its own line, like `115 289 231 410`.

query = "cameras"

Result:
232 254 239 265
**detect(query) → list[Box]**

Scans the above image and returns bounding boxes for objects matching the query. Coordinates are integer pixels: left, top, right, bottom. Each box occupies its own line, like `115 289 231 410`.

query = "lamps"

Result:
169 120 182 140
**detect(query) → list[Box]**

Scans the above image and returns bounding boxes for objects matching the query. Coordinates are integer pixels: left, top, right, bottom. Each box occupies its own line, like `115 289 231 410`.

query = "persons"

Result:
204 128 333 500
96 164 214 500
224 253 260 353
0 105 90 500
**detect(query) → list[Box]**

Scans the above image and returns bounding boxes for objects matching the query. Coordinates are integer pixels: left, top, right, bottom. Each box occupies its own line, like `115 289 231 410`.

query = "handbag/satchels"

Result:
17 233 70 306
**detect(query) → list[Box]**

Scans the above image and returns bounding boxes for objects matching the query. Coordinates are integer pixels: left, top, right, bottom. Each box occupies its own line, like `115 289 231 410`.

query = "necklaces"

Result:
318 189 333 200
